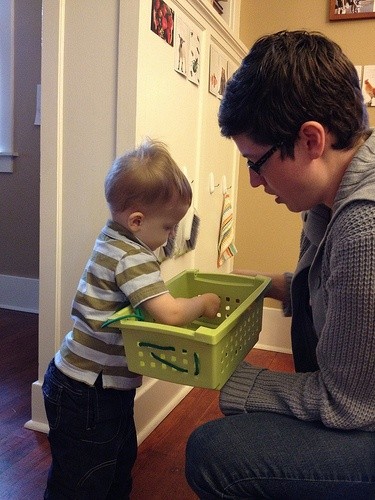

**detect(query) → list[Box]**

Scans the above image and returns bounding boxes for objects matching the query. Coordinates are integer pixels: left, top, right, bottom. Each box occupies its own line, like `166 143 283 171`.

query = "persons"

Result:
336 0 357 15
42 140 221 500
186 29 375 500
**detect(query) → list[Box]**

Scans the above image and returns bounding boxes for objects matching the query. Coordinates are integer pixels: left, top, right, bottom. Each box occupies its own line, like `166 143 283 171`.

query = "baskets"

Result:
105 269 273 390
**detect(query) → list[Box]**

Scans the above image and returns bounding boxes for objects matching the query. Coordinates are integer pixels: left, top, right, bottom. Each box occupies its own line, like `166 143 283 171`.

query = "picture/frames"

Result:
209 42 229 98
328 0 375 23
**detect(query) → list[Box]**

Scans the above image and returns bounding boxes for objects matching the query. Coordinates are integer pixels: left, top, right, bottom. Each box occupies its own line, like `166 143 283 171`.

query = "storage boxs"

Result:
106 267 273 391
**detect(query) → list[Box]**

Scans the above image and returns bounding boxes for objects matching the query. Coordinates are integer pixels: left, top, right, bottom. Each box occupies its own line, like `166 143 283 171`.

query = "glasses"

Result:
247 130 288 174
